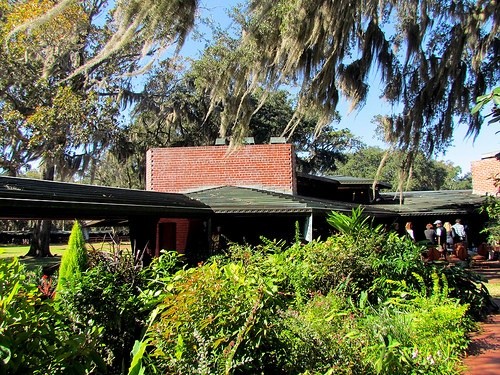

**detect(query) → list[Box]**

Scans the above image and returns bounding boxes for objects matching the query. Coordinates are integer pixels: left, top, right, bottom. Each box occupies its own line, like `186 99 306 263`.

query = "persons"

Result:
424 224 436 245
443 222 455 254
433 220 446 256
405 222 416 243
452 219 466 242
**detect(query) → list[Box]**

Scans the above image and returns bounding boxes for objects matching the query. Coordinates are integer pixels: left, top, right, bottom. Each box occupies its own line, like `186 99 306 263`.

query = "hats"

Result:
433 220 441 224
